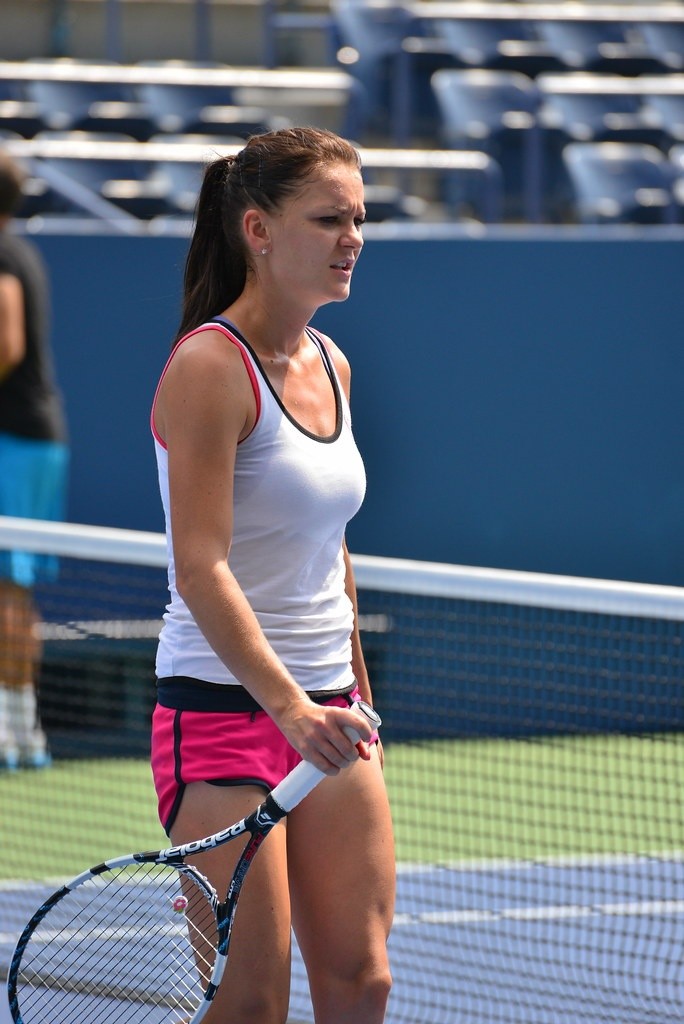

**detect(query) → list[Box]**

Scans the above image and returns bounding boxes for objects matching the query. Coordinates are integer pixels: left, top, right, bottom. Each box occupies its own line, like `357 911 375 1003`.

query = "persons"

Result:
142 125 398 1022
0 157 71 772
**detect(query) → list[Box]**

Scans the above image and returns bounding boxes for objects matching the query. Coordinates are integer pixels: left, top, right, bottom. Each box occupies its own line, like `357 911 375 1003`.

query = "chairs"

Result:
1 0 684 224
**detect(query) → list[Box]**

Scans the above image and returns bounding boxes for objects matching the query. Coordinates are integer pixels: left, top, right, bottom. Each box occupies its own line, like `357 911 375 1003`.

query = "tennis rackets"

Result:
4 699 382 1015
